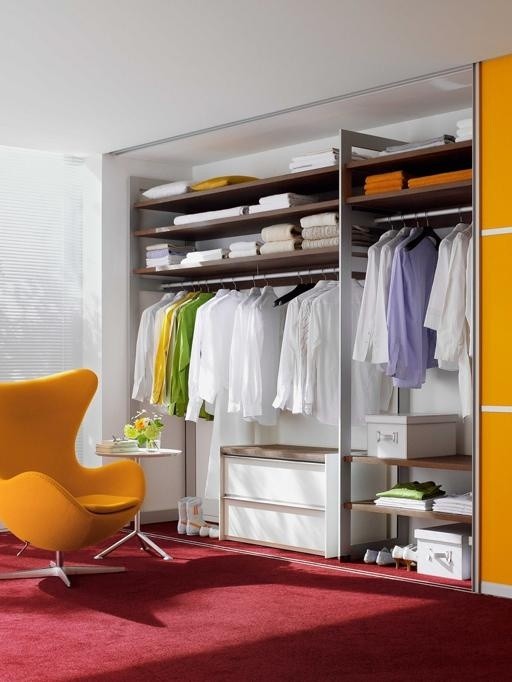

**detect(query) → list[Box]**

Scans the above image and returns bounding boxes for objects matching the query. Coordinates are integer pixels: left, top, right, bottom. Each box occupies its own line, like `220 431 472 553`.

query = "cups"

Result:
147 432 161 452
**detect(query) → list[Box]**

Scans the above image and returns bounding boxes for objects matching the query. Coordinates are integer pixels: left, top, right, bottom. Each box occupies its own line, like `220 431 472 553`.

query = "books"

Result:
96 439 139 452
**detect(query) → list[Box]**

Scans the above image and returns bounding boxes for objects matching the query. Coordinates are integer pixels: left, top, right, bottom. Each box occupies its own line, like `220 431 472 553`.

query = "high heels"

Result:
404 549 421 573
391 545 401 570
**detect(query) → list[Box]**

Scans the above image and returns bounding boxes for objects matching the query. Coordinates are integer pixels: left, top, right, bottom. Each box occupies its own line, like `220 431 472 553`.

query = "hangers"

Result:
142 279 214 316
272 265 363 309
405 211 442 253
237 271 261 308
250 273 279 310
196 277 244 312
440 207 472 247
368 211 435 252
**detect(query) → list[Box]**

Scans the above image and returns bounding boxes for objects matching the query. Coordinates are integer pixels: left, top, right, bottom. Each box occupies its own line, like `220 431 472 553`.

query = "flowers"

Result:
111 406 168 452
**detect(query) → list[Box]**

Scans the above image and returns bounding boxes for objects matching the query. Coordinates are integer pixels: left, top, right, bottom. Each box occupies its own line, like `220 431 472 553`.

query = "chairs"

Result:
0 365 149 589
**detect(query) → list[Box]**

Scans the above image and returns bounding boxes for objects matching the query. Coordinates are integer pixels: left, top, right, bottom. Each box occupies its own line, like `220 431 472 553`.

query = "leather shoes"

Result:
207 527 220 538
199 524 208 536
375 551 391 567
362 548 376 565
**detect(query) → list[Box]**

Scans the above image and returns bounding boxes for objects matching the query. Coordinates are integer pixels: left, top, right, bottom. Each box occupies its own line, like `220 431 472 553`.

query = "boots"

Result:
185 498 202 534
176 499 185 535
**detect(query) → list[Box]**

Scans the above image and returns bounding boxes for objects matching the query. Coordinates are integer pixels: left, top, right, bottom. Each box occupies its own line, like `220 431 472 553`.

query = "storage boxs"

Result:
413 522 472 580
365 413 458 459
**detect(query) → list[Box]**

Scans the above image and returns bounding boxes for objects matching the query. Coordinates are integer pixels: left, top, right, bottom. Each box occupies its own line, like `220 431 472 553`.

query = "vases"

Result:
145 431 161 452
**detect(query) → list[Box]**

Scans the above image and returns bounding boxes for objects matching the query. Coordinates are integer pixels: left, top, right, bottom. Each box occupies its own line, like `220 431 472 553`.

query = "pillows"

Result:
142 180 188 199
191 176 257 191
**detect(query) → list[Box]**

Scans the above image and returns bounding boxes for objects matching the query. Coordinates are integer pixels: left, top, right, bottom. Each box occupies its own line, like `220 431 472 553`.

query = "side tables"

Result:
90 439 183 562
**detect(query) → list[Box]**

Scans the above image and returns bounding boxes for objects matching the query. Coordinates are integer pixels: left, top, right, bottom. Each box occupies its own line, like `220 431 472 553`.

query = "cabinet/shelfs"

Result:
100 53 511 601
219 443 388 559
